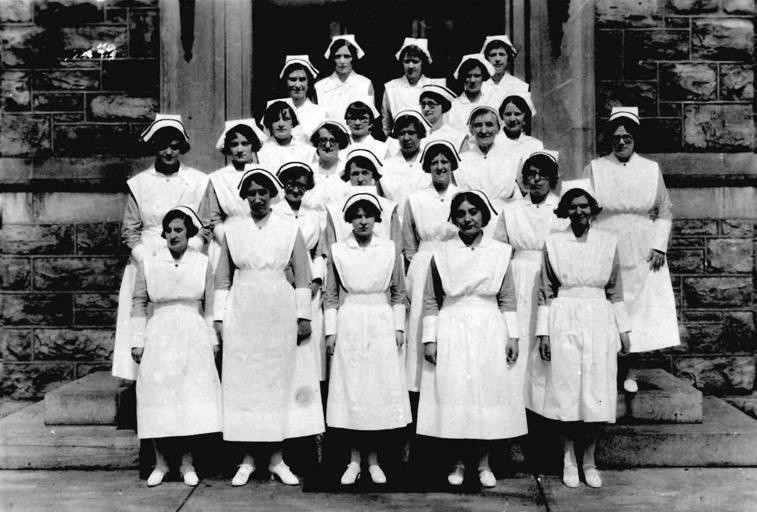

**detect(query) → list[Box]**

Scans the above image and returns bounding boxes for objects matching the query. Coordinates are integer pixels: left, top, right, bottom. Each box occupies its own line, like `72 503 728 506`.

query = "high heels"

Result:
268 461 300 488
625 371 641 393
562 459 580 490
145 458 168 484
447 460 471 483
231 460 256 485
369 460 393 485
477 463 500 488
341 458 361 488
583 460 605 489
180 460 203 487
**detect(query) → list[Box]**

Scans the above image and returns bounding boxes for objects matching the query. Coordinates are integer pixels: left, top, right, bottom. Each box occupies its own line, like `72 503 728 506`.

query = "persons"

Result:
317 35 380 129
306 119 350 199
495 92 545 152
416 188 528 487
381 37 446 137
343 100 386 155
327 149 405 277
212 164 326 486
270 160 336 382
452 105 523 203
131 205 223 487
445 53 505 130
522 177 632 489
260 54 329 144
255 98 317 177
379 107 433 200
113 114 214 382
479 34 536 117
323 191 407 483
403 141 481 262
197 119 272 274
578 106 680 394
493 149 571 415
417 83 470 154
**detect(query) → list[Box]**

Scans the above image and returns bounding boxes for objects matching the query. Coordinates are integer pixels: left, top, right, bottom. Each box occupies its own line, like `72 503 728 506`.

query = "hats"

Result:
342 96 380 120
478 35 519 61
261 94 307 122
237 164 283 197
454 54 495 84
309 118 347 138
465 102 506 127
213 119 268 148
327 35 365 58
609 106 641 125
395 38 437 64
340 144 387 178
141 113 190 141
504 93 537 118
390 111 432 135
419 85 457 103
447 188 500 219
280 55 320 83
417 137 463 164
520 151 569 171
276 161 319 179
552 178 604 213
164 204 207 229
339 192 382 214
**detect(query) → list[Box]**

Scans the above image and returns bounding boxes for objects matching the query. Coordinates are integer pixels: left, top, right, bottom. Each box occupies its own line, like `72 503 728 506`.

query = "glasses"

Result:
279 180 311 191
316 139 342 147
419 100 445 109
526 169 549 177
613 135 631 143
